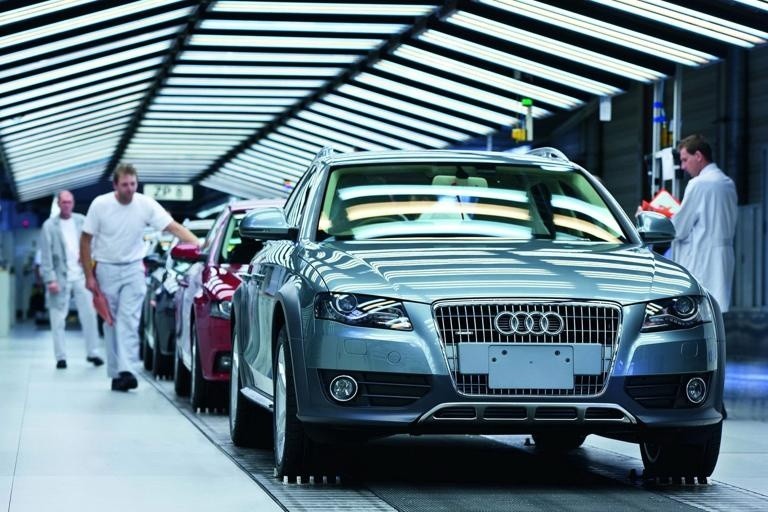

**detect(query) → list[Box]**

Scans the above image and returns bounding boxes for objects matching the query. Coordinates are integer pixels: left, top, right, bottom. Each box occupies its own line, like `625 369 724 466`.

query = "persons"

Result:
77 162 207 392
635 134 742 314
36 189 105 369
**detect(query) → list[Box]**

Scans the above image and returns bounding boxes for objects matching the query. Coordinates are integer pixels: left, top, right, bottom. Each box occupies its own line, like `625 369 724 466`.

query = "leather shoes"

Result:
57 360 68 369
87 356 105 366
112 379 127 392
118 372 138 389
